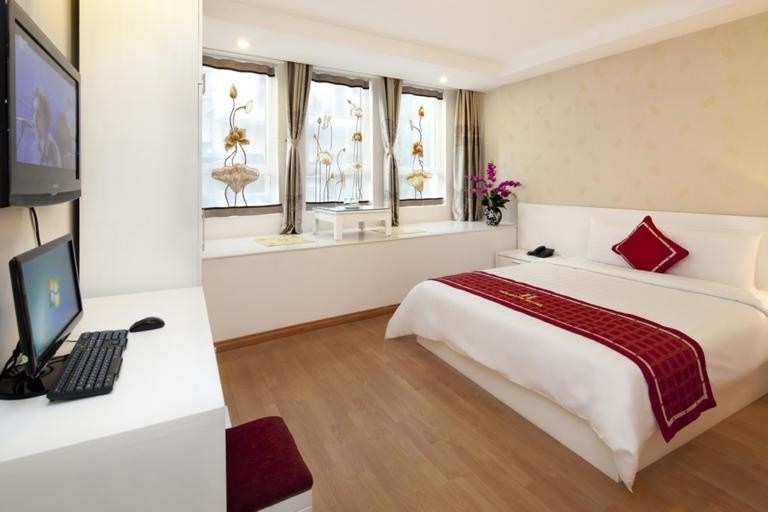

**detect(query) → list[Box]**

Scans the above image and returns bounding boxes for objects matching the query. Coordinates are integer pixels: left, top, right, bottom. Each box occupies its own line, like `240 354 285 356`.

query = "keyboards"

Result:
48 330 128 401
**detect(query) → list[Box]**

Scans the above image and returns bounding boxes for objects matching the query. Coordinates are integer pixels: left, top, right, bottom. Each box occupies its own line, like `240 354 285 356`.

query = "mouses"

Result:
130 317 164 332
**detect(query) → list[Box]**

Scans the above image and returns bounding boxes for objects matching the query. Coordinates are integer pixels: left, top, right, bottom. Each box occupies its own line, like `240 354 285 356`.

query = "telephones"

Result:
533 246 554 258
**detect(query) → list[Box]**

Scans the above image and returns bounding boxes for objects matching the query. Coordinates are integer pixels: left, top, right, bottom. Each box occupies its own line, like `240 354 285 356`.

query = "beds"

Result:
385 202 768 496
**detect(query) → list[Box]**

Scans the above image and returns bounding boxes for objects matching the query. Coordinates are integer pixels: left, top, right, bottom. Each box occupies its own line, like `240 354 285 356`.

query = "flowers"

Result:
313 98 363 203
460 160 522 210
211 83 260 207
405 106 434 198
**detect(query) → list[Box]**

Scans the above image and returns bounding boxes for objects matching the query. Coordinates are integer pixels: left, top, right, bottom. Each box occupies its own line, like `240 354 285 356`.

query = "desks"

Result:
311 205 393 241
1 285 227 511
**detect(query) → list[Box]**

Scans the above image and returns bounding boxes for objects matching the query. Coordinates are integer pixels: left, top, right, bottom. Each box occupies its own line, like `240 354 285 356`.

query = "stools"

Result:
225 417 313 512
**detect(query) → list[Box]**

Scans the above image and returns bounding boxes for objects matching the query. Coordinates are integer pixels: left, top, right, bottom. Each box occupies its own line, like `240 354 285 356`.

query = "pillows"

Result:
585 215 762 294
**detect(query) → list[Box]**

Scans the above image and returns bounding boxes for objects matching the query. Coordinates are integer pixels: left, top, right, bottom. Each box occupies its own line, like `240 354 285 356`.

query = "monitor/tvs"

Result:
0 1 82 208
0 232 84 400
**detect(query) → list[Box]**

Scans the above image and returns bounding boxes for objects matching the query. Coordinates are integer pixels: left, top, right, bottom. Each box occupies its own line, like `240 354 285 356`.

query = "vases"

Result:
483 205 502 226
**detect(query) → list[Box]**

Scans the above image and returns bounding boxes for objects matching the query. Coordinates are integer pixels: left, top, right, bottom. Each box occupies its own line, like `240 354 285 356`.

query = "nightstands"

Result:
495 246 556 266
